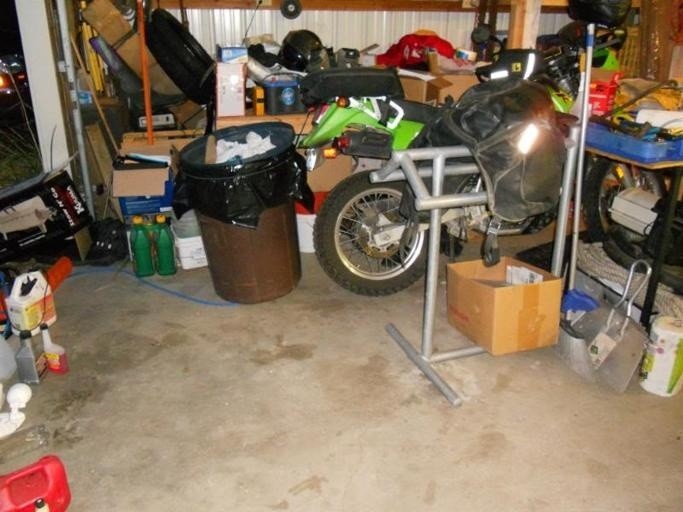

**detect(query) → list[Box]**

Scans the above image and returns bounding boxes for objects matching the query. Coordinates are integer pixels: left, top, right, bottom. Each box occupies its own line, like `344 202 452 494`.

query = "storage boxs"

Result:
445 255 562 356
587 65 682 128
0 150 93 262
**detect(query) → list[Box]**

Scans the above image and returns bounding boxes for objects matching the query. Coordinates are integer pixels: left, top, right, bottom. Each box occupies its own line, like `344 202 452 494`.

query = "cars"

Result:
0 53 29 98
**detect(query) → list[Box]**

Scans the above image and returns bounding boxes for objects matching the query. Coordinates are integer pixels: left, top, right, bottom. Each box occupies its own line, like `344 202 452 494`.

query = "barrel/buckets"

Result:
129 216 155 276
153 214 177 276
4 269 59 339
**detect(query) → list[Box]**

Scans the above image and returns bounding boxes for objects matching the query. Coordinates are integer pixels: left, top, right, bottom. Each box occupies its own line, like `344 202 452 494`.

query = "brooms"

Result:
554 22 600 383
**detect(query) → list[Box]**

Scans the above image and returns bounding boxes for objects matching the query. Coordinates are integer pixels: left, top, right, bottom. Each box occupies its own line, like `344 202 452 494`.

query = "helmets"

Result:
278 29 322 71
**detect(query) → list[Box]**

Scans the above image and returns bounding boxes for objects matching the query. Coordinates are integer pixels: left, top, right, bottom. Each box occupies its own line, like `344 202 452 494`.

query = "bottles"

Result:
76 70 93 108
639 318 682 398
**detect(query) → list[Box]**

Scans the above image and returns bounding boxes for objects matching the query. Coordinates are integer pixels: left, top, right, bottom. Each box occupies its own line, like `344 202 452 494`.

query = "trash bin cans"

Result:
178 122 301 304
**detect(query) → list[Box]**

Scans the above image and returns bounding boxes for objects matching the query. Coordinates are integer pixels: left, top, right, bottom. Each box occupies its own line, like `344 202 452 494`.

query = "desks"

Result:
560 142 681 337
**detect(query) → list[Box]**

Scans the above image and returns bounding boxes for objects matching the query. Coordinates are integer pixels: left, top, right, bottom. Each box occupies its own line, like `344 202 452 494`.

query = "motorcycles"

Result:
295 17 672 299
